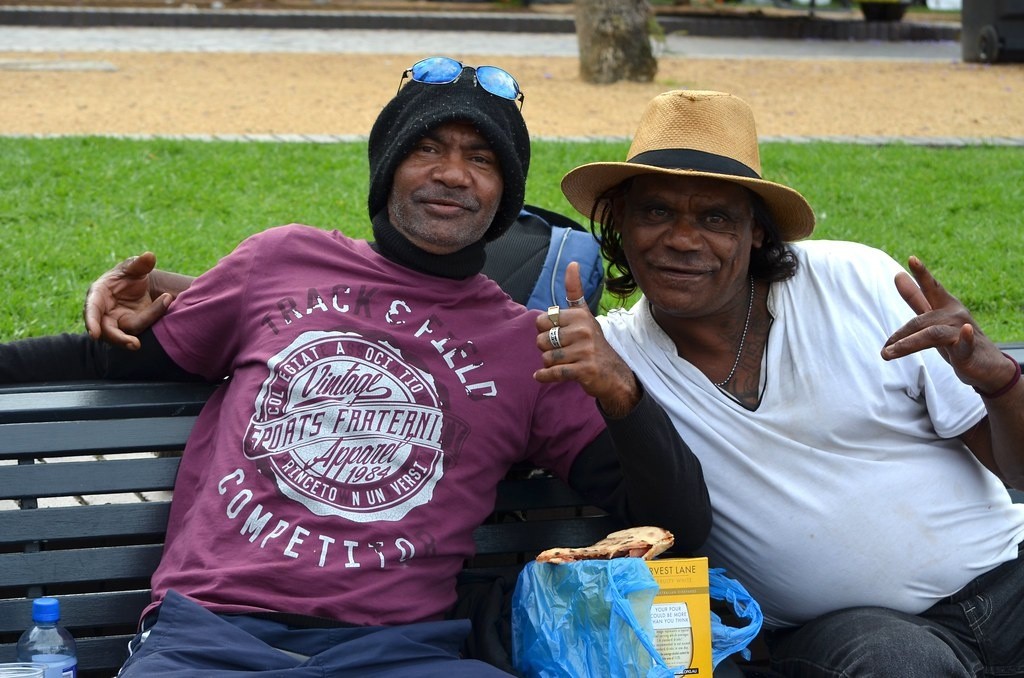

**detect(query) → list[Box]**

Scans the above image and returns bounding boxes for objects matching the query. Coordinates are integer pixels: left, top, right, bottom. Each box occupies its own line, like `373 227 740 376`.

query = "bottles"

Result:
16 597 79 678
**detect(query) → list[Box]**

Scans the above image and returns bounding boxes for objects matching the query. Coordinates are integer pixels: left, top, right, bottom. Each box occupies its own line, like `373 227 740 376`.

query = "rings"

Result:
566 297 585 307
549 326 562 348
548 305 560 325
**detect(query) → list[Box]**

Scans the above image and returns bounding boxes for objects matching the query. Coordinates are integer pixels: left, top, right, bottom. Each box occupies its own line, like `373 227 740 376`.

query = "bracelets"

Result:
973 352 1021 398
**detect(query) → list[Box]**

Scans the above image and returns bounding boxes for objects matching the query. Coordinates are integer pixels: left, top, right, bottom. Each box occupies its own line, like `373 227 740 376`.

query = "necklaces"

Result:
714 277 754 387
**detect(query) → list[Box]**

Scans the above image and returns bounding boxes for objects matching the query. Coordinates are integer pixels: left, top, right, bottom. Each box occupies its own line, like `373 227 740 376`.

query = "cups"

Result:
0 662 49 678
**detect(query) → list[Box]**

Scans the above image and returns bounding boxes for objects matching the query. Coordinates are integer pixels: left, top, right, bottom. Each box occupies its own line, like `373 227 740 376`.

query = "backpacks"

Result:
478 202 607 322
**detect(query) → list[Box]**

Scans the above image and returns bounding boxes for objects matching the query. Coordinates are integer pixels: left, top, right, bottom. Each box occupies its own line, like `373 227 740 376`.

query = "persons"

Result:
84 90 1024 678
1 57 714 678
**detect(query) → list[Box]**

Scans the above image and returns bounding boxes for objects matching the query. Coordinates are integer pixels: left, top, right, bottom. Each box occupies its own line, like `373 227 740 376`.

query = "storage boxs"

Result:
644 557 712 678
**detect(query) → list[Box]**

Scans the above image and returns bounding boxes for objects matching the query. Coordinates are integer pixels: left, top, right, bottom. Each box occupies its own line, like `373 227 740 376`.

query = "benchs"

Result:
0 342 1024 678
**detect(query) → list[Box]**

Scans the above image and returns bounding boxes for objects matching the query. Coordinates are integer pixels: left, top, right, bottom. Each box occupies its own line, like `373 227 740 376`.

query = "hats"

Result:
558 88 815 242
368 62 532 243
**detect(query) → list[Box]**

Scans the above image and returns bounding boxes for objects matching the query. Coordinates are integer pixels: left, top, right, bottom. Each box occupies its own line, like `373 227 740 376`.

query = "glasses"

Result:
398 57 525 112
